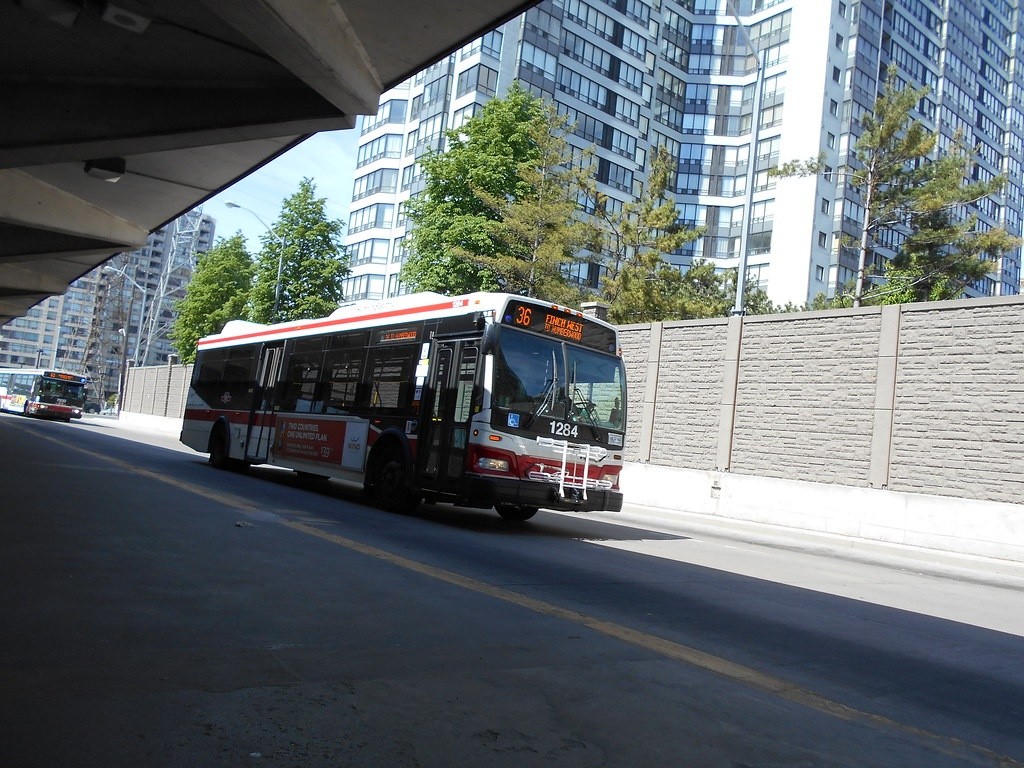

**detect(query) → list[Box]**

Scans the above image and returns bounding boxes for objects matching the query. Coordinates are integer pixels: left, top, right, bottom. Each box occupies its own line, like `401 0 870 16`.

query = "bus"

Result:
178 289 632 523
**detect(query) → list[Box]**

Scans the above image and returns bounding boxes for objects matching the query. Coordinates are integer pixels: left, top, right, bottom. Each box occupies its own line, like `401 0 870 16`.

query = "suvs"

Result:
0 368 87 422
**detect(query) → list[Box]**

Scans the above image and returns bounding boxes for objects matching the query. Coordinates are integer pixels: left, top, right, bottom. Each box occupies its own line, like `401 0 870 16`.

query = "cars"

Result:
83 402 101 415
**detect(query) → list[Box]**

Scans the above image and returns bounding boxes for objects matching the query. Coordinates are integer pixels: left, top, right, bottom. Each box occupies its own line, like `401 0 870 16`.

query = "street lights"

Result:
224 201 284 305
104 264 145 367
